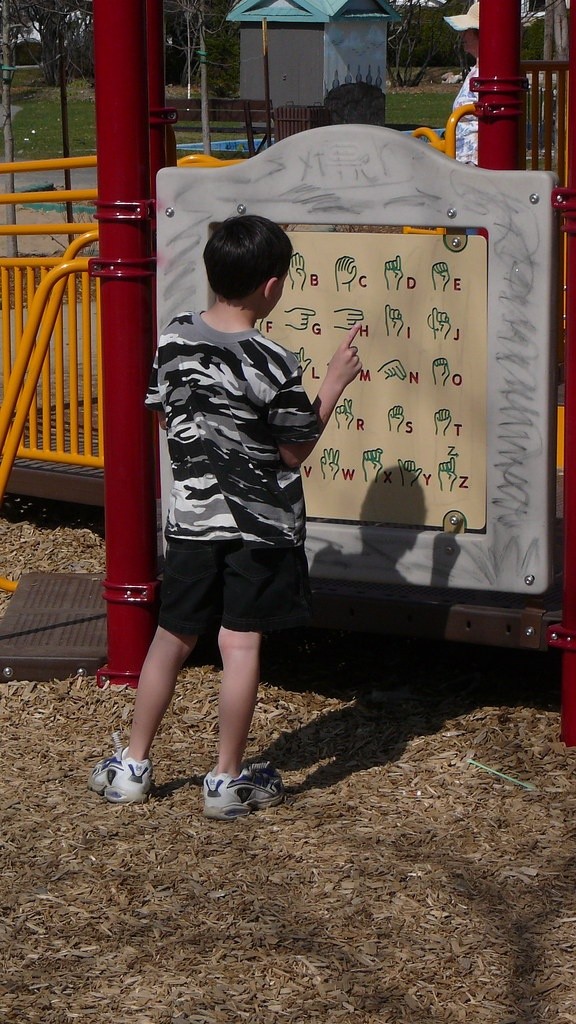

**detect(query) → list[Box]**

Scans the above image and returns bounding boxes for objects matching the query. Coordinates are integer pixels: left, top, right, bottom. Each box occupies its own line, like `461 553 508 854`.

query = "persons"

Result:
87 215 363 818
444 2 479 168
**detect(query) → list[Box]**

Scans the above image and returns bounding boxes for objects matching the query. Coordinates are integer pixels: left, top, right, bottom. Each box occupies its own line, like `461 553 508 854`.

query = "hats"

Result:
443 2 480 31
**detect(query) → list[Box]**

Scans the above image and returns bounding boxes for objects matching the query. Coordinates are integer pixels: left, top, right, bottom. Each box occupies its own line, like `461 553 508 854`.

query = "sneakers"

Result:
204 761 285 821
87 746 152 803
